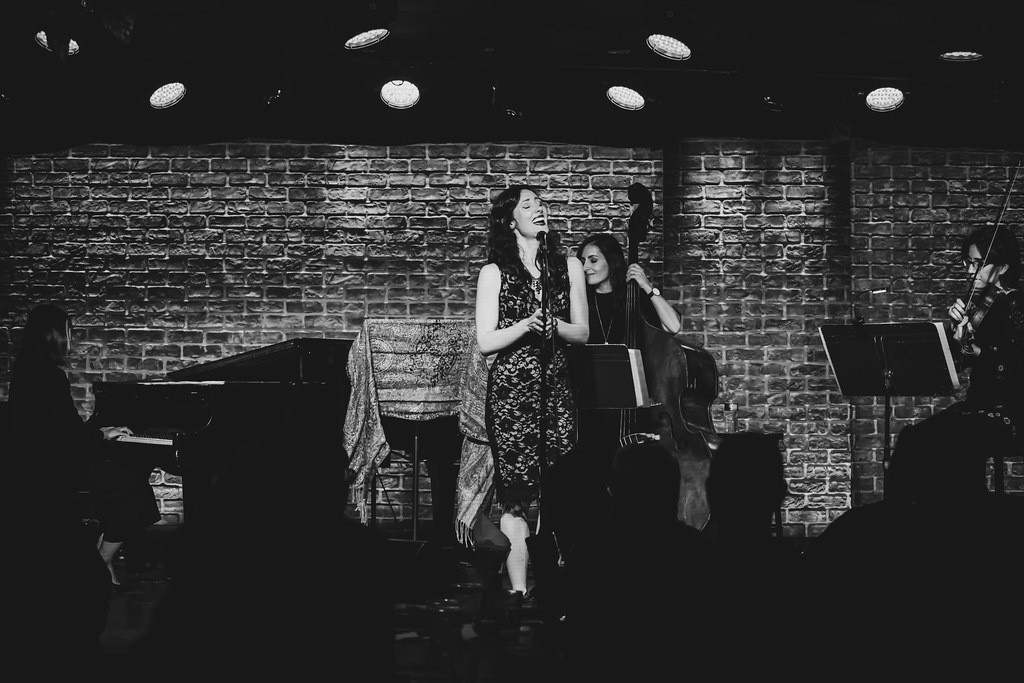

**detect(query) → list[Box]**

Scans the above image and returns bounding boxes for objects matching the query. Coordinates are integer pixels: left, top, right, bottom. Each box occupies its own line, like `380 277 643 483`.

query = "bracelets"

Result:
553 317 558 329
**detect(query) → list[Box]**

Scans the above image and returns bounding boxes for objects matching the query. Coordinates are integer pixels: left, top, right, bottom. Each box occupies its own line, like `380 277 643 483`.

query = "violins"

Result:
961 285 1003 355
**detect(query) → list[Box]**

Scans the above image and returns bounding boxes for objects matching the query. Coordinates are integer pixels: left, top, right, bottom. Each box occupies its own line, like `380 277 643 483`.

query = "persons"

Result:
476 187 590 599
886 224 1024 508
0 302 1024 683
575 233 681 485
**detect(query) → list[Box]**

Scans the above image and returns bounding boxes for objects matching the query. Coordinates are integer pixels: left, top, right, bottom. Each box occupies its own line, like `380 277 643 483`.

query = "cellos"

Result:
548 182 719 569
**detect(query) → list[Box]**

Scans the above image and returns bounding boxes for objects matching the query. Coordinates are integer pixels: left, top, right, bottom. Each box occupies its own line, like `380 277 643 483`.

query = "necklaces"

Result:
594 294 613 345
521 259 542 295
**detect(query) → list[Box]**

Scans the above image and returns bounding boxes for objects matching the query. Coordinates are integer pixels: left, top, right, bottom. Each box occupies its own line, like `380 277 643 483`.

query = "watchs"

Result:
647 287 661 299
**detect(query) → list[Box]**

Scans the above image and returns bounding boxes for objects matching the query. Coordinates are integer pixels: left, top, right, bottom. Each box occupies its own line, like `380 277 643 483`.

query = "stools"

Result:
976 435 1024 499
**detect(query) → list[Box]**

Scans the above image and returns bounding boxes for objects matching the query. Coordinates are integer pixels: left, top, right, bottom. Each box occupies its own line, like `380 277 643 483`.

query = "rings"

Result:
634 271 636 274
952 306 955 312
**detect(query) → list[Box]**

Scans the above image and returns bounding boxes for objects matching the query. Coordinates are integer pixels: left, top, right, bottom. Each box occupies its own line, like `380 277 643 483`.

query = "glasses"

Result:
962 257 992 269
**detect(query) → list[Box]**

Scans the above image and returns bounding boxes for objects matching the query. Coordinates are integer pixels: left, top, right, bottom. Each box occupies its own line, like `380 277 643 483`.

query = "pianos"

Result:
85 336 464 540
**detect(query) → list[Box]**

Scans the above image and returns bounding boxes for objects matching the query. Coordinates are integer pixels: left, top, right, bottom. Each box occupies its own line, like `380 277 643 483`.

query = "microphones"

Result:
536 230 548 241
